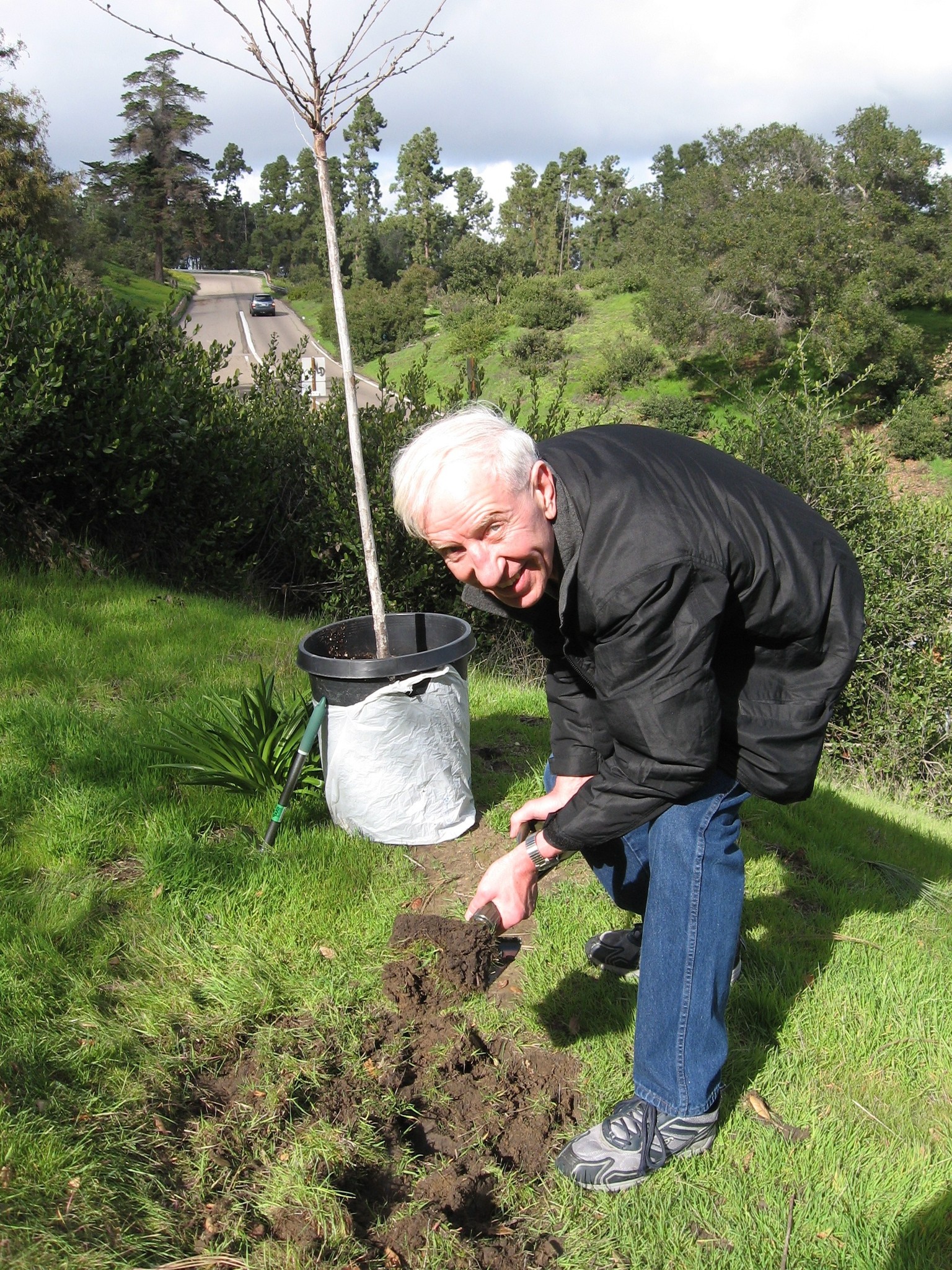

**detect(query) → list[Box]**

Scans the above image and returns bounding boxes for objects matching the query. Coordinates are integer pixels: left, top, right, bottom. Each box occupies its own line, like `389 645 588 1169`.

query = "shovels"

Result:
386 821 579 1014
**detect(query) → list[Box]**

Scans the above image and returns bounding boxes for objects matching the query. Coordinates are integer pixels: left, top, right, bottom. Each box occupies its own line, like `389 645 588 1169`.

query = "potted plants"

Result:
87 0 484 850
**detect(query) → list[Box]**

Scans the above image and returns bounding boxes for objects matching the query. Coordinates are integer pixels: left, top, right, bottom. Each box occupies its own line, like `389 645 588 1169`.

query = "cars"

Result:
249 293 276 316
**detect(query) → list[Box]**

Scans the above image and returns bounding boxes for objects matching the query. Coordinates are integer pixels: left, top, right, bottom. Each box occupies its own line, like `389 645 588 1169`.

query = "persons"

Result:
387 393 864 1191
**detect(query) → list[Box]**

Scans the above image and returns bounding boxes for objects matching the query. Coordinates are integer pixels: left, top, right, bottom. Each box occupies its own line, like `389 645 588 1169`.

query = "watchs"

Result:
525 828 561 873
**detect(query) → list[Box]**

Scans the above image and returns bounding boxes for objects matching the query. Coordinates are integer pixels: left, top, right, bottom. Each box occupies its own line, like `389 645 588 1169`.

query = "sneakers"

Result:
555 1094 720 1190
586 923 742 982
